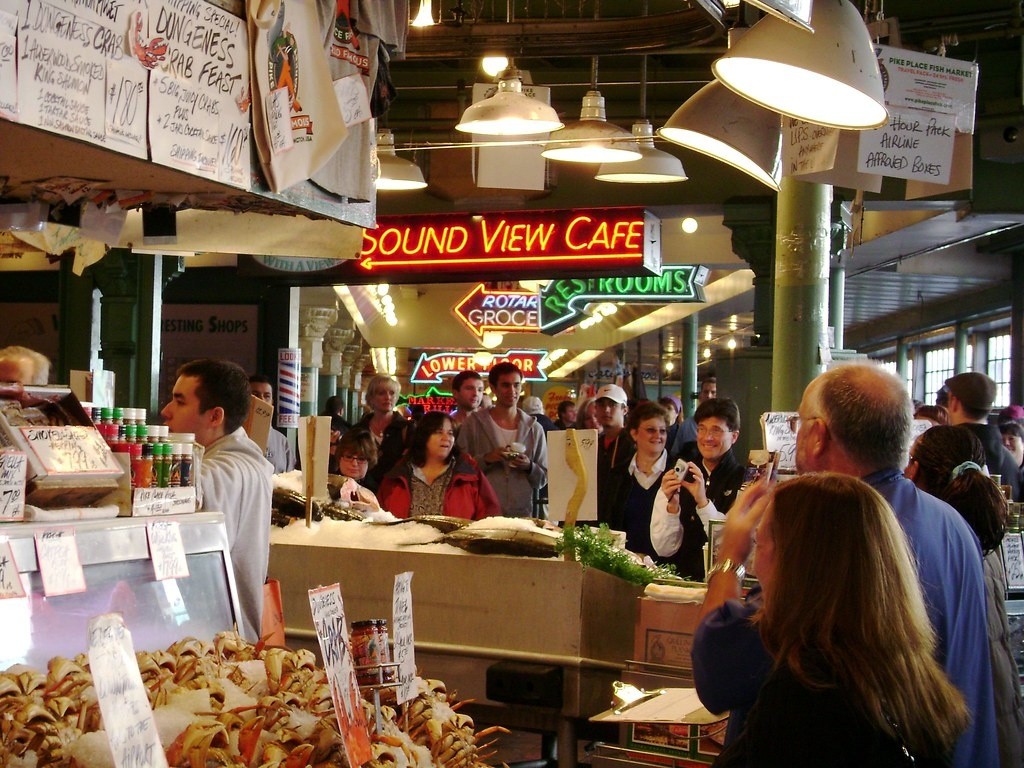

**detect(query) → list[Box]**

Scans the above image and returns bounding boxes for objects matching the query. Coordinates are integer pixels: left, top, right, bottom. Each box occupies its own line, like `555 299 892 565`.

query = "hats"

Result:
522 397 544 415
595 384 627 406
1000 406 1024 419
946 371 996 411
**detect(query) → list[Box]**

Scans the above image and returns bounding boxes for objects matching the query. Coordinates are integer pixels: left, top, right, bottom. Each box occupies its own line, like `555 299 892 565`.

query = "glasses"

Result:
341 454 369 464
696 426 732 437
786 414 831 441
637 426 666 434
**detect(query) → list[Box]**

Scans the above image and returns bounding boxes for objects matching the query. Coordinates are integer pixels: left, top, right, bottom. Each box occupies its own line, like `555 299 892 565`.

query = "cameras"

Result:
674 459 689 484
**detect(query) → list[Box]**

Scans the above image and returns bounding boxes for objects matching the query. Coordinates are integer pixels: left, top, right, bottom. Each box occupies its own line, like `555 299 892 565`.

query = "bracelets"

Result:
705 558 746 587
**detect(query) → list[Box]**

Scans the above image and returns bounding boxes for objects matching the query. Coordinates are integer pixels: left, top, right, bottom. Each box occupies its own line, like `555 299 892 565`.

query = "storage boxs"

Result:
621 584 734 759
241 394 274 455
1 381 123 509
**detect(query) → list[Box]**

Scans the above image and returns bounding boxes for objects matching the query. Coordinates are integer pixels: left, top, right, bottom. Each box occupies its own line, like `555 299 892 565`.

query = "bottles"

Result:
351 618 395 685
83 406 194 502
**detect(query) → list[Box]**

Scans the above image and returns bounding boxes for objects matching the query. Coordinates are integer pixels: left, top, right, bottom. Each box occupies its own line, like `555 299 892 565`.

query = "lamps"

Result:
367 0 887 189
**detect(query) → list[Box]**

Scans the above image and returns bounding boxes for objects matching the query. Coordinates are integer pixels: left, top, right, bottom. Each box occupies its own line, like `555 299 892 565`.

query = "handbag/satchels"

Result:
260 577 285 648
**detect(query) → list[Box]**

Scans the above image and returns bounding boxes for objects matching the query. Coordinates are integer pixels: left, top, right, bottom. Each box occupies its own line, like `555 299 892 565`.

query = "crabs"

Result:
0 621 512 768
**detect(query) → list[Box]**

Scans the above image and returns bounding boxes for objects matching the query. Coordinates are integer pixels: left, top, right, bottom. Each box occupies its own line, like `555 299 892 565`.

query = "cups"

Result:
508 443 526 468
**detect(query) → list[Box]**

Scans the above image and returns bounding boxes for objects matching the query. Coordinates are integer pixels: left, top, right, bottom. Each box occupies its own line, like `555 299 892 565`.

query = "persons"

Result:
238 362 754 585
160 358 275 642
692 474 963 768
900 373 1024 768
793 364 991 768
